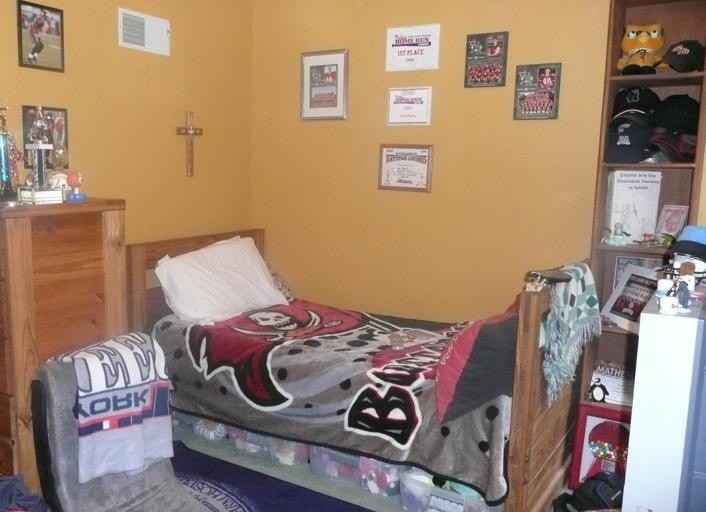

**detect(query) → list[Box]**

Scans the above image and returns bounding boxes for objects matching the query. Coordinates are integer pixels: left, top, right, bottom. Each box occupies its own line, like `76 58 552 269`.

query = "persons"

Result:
467 35 503 83
309 70 337 103
658 213 681 244
517 67 555 114
27 10 50 65
614 294 645 317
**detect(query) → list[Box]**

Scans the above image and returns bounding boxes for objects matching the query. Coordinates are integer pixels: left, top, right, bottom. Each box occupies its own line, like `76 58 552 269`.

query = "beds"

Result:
126 227 590 512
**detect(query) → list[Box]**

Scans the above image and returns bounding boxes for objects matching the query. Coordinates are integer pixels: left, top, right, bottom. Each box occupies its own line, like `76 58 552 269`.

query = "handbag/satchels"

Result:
551 471 624 512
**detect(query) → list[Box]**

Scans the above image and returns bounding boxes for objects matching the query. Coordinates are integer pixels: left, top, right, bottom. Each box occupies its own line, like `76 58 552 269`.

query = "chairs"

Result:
33 360 213 511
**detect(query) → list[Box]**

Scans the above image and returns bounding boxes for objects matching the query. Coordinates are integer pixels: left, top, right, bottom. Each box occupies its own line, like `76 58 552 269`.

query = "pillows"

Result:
264 260 294 303
154 234 290 327
430 305 517 428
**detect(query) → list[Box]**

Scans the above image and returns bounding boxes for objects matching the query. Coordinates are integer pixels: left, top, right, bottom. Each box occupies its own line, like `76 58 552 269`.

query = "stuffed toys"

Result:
615 22 668 75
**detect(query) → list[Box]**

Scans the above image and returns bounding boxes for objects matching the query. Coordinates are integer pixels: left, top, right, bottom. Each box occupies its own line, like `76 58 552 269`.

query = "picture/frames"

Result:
514 61 561 121
566 403 631 492
299 48 349 121
385 84 433 127
383 23 441 73
16 0 66 74
21 104 69 169
378 143 434 193
463 30 508 88
599 264 661 338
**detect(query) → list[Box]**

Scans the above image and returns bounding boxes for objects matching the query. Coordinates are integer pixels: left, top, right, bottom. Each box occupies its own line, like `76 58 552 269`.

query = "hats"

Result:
653 39 706 73
670 224 706 261
603 85 699 167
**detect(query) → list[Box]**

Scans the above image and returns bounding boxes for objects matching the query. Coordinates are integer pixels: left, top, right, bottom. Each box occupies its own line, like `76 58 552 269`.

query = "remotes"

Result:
390 334 404 350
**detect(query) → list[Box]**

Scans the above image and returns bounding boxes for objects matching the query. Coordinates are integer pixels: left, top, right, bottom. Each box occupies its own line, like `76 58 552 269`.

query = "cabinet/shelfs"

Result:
0 193 126 499
579 0 706 416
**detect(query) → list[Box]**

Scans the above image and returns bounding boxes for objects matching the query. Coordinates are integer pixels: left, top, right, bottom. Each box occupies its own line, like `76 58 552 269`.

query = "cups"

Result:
655 278 675 297
658 296 679 315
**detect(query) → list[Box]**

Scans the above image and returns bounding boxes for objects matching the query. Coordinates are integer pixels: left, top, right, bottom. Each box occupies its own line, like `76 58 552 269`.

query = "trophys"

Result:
0 106 23 202
17 104 64 205
